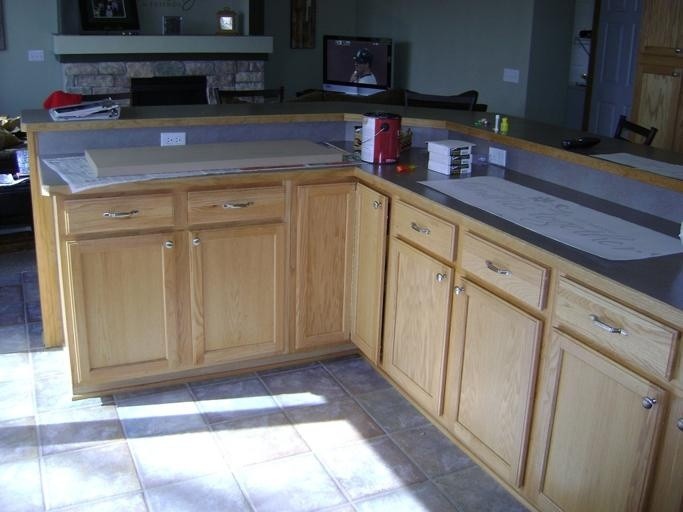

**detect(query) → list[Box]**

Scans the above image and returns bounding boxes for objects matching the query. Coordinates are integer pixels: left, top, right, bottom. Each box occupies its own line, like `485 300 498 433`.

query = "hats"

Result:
44 91 82 108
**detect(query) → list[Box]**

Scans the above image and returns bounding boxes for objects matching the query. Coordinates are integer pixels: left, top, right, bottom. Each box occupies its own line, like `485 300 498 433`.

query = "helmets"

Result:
352 48 370 64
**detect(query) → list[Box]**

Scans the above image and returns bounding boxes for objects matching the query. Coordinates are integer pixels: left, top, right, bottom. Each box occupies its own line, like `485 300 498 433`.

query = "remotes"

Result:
562 136 602 149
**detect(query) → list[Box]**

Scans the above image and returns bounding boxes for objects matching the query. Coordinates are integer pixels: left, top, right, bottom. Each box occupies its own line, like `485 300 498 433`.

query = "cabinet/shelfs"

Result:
61 177 291 388
377 196 559 497
520 254 681 510
293 179 390 366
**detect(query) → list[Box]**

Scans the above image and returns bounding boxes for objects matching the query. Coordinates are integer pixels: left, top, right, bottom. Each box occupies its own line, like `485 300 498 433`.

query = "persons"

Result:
350 47 377 84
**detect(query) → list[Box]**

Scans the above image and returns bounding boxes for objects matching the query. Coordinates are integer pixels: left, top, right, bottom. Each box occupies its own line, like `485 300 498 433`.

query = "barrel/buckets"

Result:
360 112 402 164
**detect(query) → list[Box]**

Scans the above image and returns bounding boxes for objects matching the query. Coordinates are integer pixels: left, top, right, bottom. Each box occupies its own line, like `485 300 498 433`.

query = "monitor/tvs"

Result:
323 35 395 96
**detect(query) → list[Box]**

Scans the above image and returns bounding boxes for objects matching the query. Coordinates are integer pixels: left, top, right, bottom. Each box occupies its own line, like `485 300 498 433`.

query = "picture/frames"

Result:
76 1 141 36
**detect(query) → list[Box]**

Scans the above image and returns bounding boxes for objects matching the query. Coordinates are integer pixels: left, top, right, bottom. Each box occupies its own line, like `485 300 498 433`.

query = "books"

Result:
50 100 120 120
